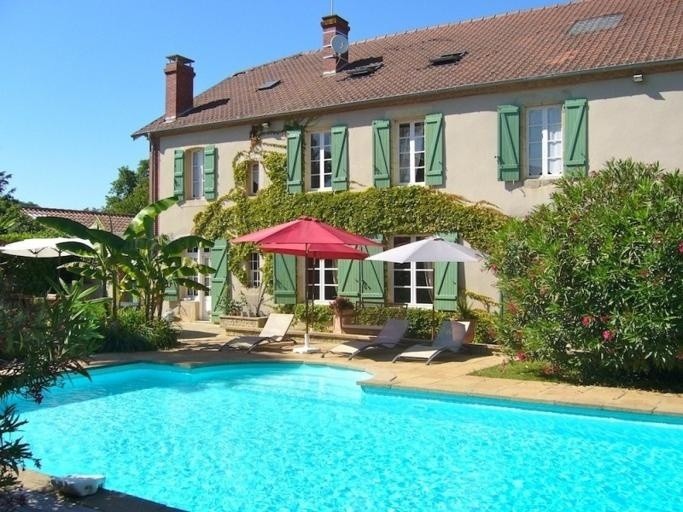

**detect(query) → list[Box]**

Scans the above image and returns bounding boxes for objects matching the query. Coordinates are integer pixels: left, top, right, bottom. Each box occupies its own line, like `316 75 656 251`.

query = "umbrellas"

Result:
258 243 367 331
0 238 95 277
232 216 386 348
364 237 484 341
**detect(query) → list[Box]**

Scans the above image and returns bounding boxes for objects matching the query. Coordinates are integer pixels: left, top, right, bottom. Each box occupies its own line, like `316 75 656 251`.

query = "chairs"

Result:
219 313 471 364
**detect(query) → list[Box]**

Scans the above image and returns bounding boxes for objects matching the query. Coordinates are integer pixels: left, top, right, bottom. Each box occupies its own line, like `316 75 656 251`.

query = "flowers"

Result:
329 297 353 314
